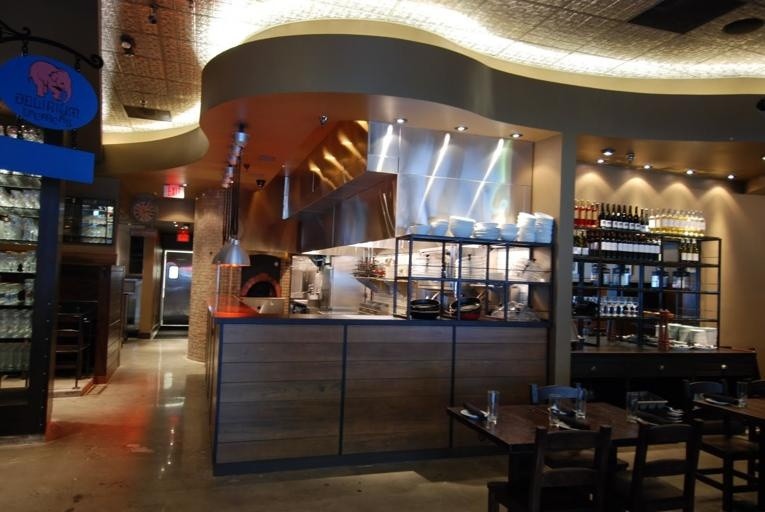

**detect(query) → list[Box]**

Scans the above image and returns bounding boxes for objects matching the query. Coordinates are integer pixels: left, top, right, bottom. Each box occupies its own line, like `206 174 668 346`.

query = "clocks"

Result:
130 197 160 224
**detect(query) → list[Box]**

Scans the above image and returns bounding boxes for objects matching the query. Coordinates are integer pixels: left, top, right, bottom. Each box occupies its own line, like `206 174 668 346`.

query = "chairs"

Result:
486 376 765 512
54 310 93 390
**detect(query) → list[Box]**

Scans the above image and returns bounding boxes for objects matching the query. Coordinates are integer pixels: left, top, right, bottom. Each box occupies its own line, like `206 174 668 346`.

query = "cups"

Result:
487 389 501 423
547 393 562 430
574 388 588 420
736 381 748 409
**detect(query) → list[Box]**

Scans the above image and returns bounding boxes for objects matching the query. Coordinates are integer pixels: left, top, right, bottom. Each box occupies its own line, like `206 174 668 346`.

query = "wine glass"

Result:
599 295 639 317
0 248 29 306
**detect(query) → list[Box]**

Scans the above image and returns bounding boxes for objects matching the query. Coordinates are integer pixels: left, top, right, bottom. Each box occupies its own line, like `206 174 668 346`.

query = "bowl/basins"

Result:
408 211 555 245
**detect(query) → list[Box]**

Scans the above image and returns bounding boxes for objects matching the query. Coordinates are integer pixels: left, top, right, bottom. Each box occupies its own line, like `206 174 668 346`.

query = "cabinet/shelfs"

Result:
571 226 722 349
392 233 556 326
0 114 65 436
572 349 756 407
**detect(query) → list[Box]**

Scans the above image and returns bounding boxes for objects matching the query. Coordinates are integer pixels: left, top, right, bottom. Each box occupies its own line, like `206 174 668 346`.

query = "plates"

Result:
460 408 489 418
667 323 717 350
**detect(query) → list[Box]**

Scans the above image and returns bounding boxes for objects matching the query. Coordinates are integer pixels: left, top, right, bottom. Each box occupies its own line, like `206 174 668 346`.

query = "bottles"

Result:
590 263 691 292
572 198 706 263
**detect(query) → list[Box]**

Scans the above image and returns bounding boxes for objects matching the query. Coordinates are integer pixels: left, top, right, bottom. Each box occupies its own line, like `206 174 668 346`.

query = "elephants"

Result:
27 61 72 103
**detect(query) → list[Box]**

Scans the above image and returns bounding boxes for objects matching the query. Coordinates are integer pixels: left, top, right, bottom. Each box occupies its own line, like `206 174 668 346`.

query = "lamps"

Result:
210 121 252 270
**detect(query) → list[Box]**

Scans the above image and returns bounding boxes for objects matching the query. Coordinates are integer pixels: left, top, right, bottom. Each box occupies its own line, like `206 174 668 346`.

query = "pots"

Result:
449 288 488 319
408 289 441 320
